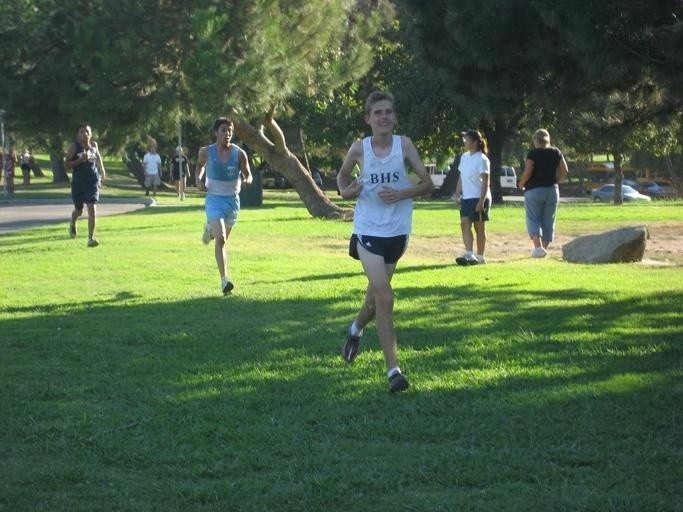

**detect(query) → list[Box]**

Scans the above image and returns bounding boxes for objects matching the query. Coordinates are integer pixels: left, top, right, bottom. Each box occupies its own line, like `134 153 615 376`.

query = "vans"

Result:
424 165 450 193
499 165 517 193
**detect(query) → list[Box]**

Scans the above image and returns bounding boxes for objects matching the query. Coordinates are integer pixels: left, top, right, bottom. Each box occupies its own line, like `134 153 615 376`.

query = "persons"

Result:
141 143 162 196
516 129 569 259
169 146 190 201
64 124 105 247
452 128 490 265
314 172 322 186
0 149 33 196
193 116 253 296
335 90 434 394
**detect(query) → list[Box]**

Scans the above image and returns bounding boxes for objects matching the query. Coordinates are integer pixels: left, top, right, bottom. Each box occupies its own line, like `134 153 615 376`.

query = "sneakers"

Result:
202 223 212 245
532 247 547 257
70 222 78 238
88 239 98 246
221 279 234 294
342 321 363 362
456 255 486 265
388 373 410 393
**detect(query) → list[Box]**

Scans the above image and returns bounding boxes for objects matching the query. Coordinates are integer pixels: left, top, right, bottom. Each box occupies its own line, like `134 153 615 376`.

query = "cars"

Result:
592 184 652 203
615 178 639 191
259 165 321 189
640 181 678 198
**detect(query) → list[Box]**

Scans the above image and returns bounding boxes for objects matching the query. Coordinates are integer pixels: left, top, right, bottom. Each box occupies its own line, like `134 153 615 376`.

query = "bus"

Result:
584 162 672 194
